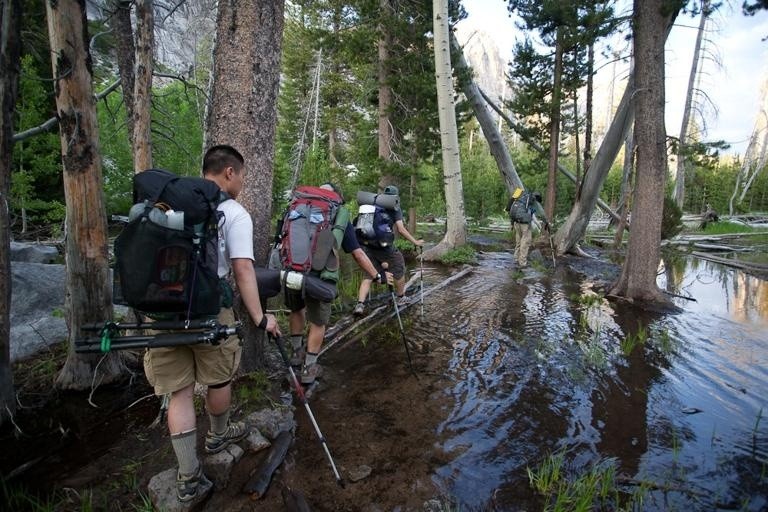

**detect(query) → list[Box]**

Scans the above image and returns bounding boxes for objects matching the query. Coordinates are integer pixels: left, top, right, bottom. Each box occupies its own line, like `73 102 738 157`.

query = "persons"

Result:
352 185 425 315
270 178 394 384
140 144 282 503
510 191 551 269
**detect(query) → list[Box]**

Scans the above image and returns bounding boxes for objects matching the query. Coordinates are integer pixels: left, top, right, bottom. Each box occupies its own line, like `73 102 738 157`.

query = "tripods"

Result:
75 318 245 354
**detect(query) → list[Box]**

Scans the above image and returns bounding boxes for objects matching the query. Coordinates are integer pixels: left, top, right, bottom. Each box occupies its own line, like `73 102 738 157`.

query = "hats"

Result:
384 185 398 195
534 192 542 202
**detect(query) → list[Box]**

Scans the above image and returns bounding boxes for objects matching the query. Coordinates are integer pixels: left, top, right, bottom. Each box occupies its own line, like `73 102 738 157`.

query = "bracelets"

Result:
255 314 268 331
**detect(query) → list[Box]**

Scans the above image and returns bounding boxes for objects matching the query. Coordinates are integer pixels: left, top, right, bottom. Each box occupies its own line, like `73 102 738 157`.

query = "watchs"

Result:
373 272 382 282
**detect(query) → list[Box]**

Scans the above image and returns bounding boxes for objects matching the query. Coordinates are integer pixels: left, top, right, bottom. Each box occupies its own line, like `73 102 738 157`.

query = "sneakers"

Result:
397 296 411 305
301 363 322 383
290 341 306 366
204 421 249 455
352 303 364 314
177 459 203 501
514 258 531 268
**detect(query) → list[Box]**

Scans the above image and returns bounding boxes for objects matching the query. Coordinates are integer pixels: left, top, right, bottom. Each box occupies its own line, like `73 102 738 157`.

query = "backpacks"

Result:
280 186 342 276
109 168 232 316
510 193 534 224
355 203 394 248
